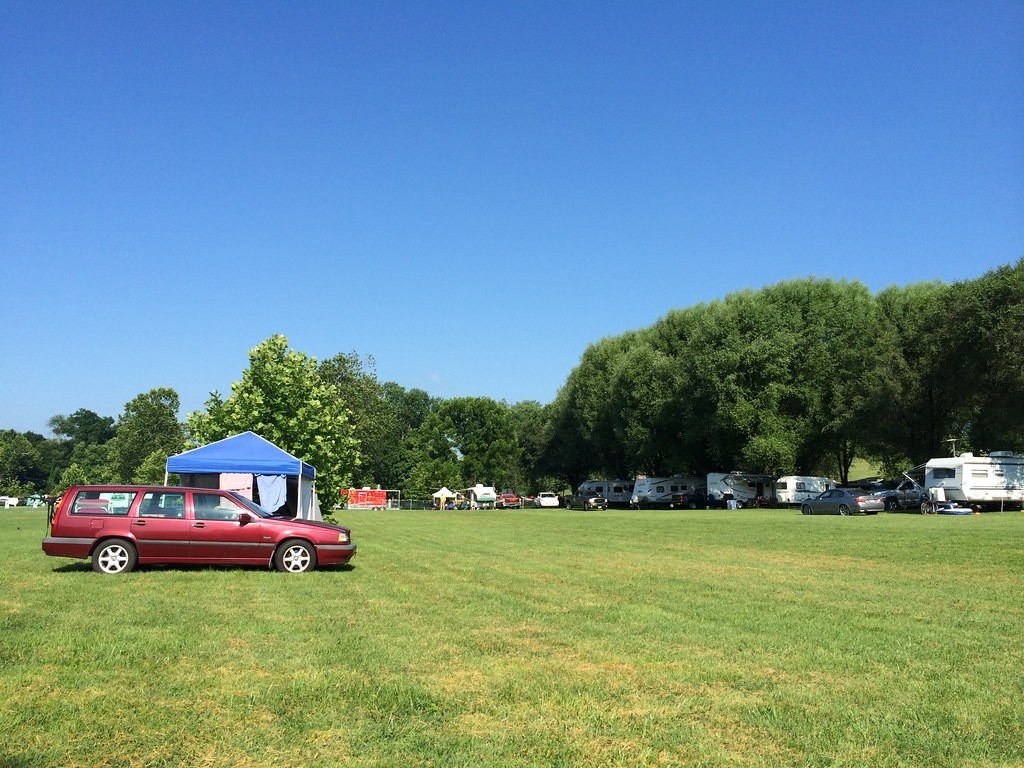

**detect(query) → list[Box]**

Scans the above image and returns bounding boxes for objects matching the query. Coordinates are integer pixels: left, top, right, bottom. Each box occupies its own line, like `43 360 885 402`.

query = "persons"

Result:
440 494 446 510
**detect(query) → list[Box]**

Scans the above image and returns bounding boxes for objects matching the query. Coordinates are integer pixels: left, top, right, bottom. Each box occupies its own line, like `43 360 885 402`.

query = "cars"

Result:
802 487 884 516
866 480 921 511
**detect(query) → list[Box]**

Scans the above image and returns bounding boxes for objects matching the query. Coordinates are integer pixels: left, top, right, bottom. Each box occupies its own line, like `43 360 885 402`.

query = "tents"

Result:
433 486 457 505
161 431 323 522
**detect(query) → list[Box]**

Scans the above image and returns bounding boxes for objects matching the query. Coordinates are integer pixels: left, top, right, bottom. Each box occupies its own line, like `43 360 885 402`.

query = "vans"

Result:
775 475 836 509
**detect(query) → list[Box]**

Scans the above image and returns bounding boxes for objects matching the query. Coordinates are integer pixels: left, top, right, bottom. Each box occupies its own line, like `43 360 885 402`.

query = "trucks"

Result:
630 473 707 510
574 480 635 506
924 450 1024 511
707 470 778 509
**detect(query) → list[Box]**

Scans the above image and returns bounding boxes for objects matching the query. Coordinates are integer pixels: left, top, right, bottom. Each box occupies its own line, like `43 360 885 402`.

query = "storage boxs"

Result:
929 487 945 501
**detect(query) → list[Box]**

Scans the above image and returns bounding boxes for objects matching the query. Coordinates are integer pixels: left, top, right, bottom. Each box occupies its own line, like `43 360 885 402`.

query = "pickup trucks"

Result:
496 494 522 509
42 484 356 574
564 489 607 511
534 492 559 508
